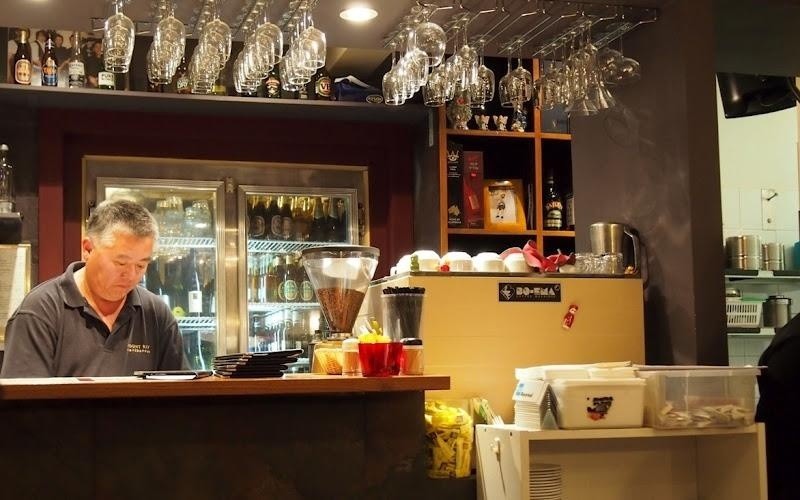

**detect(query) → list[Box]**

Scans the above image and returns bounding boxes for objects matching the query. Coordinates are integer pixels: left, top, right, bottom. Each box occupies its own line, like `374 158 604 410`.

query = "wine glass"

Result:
102 0 644 121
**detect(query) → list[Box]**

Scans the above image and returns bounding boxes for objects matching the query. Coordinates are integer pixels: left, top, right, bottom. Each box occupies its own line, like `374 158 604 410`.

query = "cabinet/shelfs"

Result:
411 55 575 257
724 266 799 338
93 174 359 374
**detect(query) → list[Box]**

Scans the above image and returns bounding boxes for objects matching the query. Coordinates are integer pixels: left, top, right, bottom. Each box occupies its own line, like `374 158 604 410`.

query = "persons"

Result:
0 198 194 378
754 311 800 500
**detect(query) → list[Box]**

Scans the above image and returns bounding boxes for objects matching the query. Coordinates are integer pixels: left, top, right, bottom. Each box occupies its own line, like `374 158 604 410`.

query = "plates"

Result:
529 462 563 500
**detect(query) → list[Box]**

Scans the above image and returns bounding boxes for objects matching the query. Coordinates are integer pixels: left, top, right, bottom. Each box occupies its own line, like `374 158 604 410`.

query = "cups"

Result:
587 221 642 277
389 250 535 277
153 196 213 238
574 251 623 275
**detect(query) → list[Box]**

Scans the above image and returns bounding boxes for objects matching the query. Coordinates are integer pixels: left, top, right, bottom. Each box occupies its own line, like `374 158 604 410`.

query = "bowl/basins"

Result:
357 341 403 378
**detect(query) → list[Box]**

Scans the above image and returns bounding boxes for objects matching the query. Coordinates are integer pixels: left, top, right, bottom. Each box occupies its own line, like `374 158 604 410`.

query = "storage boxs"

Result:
550 378 647 430
635 368 763 430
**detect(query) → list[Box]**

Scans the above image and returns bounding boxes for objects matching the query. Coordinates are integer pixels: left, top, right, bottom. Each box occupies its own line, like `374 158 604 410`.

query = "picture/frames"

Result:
483 179 526 232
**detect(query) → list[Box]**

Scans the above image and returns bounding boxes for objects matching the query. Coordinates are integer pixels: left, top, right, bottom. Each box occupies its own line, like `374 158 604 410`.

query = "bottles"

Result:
0 143 18 214
182 327 208 370
11 27 133 90
145 247 216 318
245 194 347 357
342 337 362 379
401 336 425 375
543 168 563 230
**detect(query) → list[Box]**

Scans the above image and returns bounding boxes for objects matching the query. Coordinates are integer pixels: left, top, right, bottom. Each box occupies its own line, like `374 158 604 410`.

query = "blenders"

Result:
299 243 380 373
